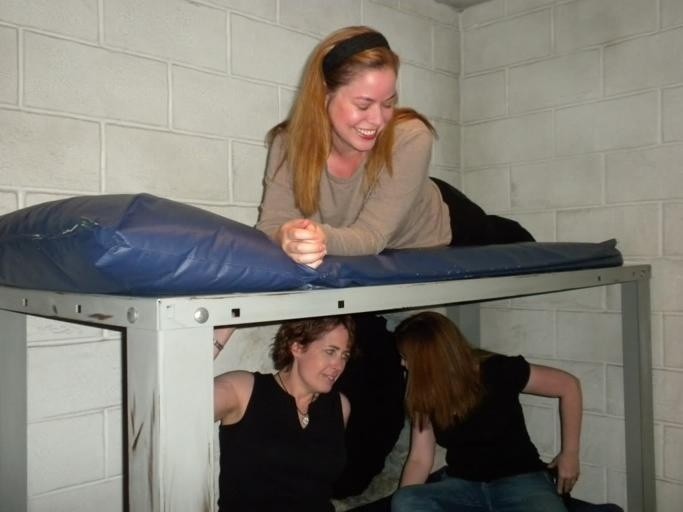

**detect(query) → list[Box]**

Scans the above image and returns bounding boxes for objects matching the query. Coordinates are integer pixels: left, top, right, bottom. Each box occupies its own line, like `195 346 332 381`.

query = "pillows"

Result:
1 194 318 293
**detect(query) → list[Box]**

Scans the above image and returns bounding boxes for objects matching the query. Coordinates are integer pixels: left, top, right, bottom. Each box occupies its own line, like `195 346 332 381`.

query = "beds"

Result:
0 263 657 511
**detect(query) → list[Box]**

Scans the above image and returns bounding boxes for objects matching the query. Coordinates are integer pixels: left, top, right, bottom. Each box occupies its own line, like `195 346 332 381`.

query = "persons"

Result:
212 315 352 512
331 313 407 502
392 312 582 512
255 25 537 270
